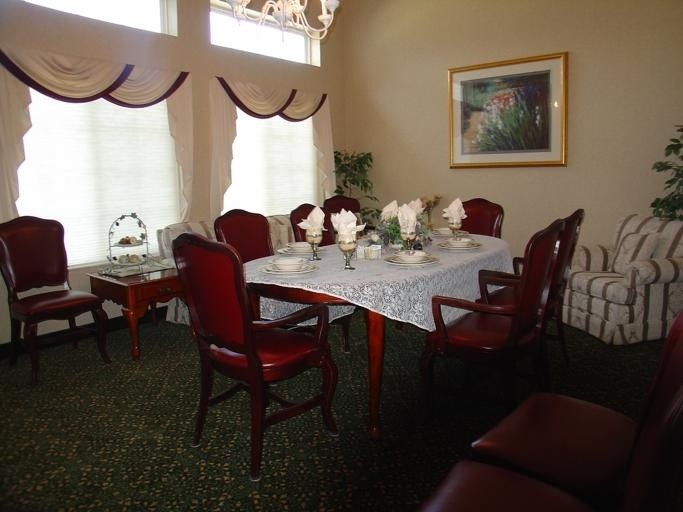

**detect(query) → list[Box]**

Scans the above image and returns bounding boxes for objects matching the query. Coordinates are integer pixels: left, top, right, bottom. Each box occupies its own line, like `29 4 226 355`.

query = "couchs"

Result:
156 214 295 323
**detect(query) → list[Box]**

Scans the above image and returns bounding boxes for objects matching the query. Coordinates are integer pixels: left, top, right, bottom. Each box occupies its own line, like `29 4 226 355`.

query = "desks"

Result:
242 227 510 435
86 261 180 360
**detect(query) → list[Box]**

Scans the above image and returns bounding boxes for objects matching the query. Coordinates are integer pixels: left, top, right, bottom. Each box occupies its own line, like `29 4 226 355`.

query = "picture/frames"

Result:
446 49 570 168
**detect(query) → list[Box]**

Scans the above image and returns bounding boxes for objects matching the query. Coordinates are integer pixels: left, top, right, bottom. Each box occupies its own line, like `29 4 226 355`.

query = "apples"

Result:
119 253 139 263
120 236 136 244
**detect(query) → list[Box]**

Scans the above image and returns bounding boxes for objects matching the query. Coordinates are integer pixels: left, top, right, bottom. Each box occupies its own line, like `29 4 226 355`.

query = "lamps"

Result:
226 0 341 41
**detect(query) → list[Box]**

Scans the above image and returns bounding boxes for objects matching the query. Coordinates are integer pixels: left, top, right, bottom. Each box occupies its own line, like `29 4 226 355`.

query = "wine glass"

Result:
336 232 358 270
306 227 321 261
448 216 463 241
398 224 417 255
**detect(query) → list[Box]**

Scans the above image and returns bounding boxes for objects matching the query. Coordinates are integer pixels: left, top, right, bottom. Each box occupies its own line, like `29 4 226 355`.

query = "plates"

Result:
435 242 484 251
274 247 320 255
383 256 440 266
115 258 146 266
114 240 144 246
259 264 320 274
431 231 468 236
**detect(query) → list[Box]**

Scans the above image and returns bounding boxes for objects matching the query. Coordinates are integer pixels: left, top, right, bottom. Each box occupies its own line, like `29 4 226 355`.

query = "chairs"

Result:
171 233 340 481
1 216 111 381
564 211 682 347
290 194 361 248
475 207 584 368
456 198 504 239
422 311 682 512
424 216 564 403
214 208 352 356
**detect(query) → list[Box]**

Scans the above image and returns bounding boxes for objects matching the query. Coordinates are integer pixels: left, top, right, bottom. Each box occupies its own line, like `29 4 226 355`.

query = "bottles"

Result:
364 245 381 259
413 232 433 249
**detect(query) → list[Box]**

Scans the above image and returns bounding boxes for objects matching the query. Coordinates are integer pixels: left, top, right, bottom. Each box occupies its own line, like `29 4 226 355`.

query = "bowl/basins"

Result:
268 257 311 269
445 237 474 246
286 241 312 250
394 249 430 262
